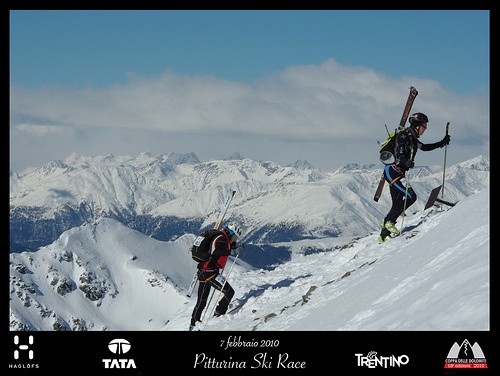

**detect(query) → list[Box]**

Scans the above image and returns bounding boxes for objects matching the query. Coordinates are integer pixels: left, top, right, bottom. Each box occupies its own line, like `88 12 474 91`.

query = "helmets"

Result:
409 112 428 127
227 221 243 239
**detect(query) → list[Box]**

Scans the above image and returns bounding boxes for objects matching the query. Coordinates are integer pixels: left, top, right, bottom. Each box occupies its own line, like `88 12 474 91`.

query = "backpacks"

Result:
190 228 225 262
380 126 412 165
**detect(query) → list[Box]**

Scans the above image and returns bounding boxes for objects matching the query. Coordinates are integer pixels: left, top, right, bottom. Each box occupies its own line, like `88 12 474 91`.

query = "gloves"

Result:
231 246 245 256
440 135 451 148
402 159 415 168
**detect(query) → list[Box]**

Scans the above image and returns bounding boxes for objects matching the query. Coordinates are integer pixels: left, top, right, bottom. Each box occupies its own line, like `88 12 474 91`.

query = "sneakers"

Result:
380 218 401 235
378 235 393 244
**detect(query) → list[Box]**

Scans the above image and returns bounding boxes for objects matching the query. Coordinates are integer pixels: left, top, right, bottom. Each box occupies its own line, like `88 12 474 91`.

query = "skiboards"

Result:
373 84 418 202
186 190 236 297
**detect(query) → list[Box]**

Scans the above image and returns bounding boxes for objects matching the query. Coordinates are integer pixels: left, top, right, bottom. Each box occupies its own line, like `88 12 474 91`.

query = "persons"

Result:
380 112 449 241
189 221 242 332
